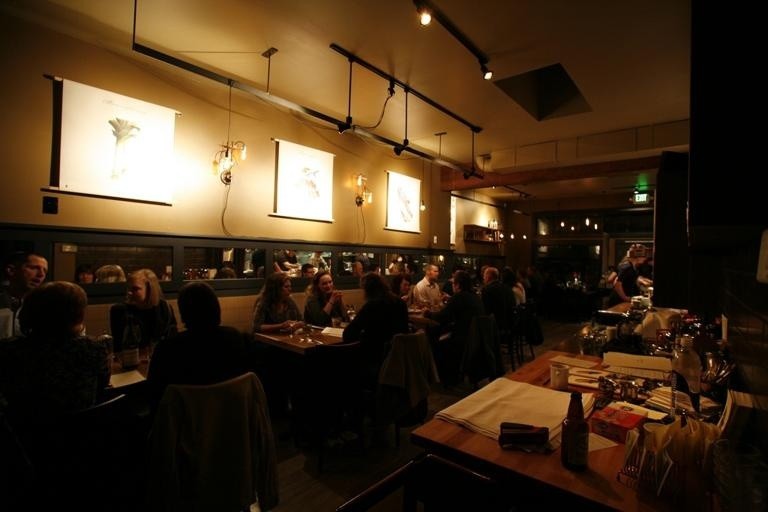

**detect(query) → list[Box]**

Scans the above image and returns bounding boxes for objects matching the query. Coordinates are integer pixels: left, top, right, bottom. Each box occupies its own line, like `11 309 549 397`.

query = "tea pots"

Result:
615 311 645 340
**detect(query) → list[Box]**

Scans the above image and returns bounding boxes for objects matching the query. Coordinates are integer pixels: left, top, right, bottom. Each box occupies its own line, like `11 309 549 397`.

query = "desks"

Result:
410 347 715 511
601 300 687 334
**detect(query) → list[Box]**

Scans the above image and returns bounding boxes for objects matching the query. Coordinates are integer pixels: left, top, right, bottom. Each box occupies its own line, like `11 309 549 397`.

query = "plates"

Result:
408 308 421 313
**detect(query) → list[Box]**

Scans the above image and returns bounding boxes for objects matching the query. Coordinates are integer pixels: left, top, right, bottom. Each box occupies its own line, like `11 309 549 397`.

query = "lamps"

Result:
217 140 246 184
355 171 374 208
407 1 493 81
327 41 483 179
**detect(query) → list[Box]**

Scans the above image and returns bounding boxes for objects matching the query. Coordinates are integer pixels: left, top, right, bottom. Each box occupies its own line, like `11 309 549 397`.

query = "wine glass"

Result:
285 307 300 339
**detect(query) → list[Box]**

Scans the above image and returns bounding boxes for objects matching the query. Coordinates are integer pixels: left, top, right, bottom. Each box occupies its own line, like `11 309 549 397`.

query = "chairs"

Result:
2 255 550 511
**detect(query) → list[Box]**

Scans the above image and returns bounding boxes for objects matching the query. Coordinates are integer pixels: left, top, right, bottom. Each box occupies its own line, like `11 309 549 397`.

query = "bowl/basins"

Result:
618 374 645 398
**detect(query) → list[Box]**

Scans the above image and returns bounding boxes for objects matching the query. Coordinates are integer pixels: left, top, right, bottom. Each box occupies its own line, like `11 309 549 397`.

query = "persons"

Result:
607 243 655 307
0 247 543 512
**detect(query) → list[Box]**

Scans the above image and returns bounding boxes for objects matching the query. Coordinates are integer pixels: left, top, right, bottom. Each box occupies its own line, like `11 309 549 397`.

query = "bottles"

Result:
96 313 140 378
673 337 702 411
561 389 591 472
185 268 209 280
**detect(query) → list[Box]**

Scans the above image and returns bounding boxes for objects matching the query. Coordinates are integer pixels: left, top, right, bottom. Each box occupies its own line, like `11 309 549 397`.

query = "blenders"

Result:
651 313 683 356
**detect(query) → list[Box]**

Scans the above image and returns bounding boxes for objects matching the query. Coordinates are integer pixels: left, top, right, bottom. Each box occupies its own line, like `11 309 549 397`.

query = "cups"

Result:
710 439 757 511
1 308 15 339
641 420 672 452
550 363 570 395
